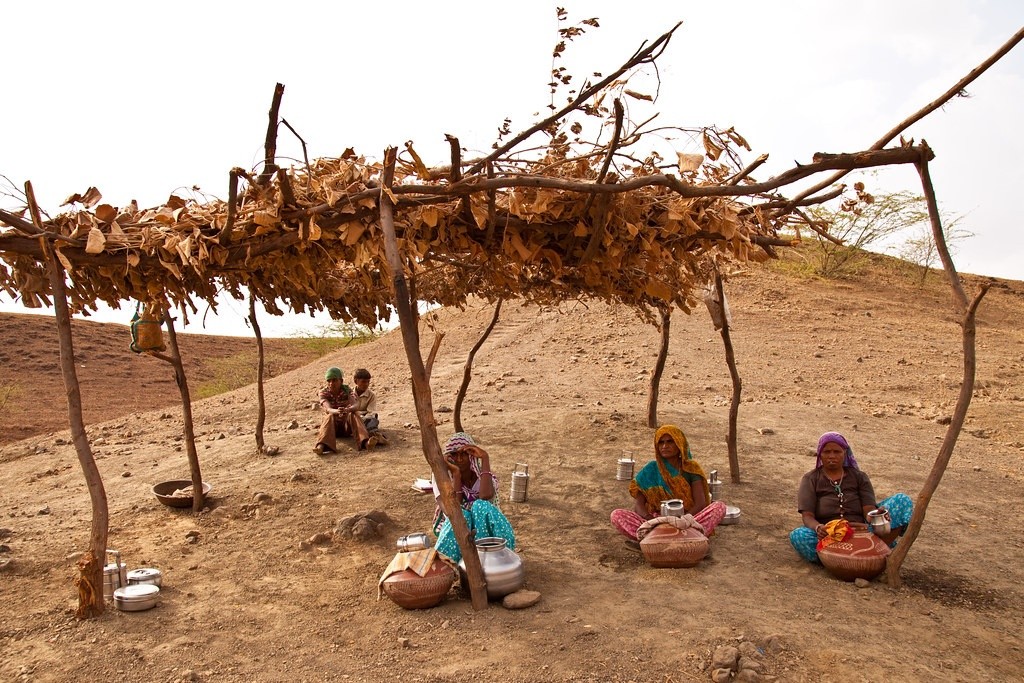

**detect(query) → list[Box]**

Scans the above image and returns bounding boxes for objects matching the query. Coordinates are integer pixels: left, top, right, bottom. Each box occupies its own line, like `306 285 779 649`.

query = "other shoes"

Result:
624 540 642 553
704 549 717 565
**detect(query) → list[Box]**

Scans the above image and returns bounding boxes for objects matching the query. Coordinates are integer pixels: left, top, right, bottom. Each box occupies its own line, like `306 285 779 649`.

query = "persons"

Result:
611 425 727 541
350 368 387 445
790 432 913 564
312 367 379 455
431 432 516 563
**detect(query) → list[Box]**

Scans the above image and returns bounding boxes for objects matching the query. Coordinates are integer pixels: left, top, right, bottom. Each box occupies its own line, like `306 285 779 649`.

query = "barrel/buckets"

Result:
615 450 635 479
510 462 529 503
103 550 127 600
706 469 721 502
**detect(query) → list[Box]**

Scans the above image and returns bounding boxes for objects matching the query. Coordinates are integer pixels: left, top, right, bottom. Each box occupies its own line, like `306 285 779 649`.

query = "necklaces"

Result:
821 468 844 495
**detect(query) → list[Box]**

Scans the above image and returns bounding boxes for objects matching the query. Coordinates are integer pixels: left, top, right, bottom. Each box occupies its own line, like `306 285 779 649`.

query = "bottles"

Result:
396 532 430 551
868 509 892 537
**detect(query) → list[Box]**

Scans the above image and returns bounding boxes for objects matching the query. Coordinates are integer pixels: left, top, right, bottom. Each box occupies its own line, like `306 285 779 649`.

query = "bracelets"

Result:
815 524 824 533
480 472 492 476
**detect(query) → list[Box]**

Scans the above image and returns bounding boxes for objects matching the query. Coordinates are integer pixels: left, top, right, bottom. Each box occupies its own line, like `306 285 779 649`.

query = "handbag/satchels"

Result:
129 300 166 353
